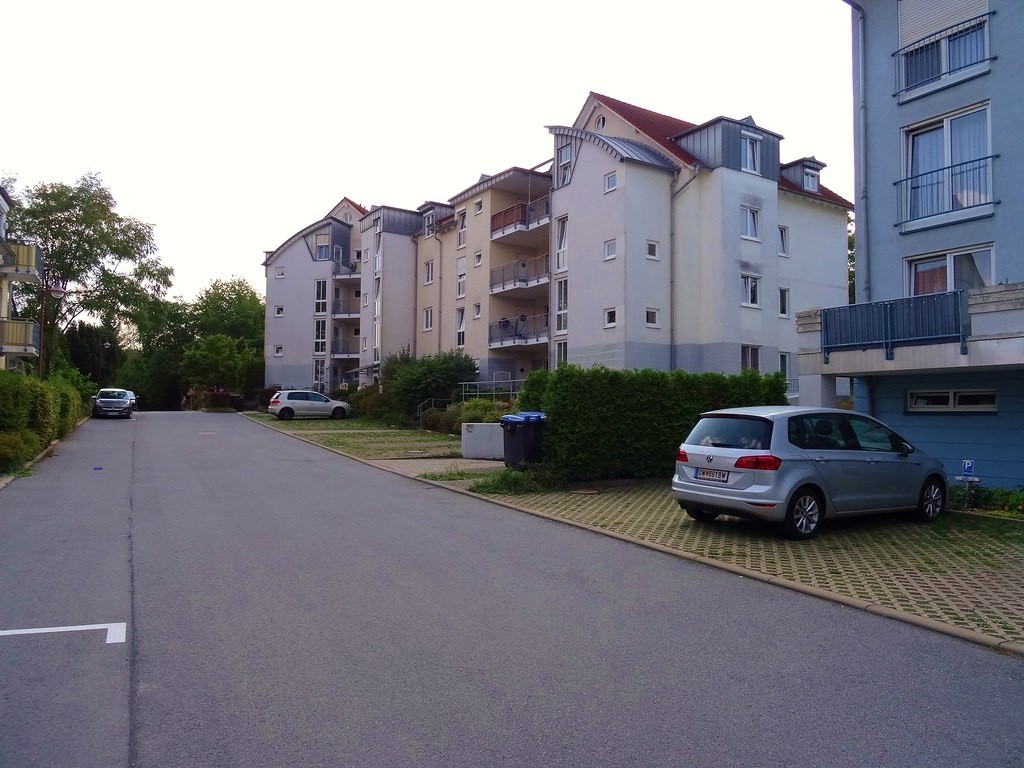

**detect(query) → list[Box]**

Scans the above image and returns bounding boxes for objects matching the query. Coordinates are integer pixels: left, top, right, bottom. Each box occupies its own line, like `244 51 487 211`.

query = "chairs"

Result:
808 420 841 448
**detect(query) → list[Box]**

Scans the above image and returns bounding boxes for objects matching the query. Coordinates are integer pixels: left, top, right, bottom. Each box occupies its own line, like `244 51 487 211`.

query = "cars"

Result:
126 391 140 410
91 388 134 418
670 400 948 538
268 390 353 420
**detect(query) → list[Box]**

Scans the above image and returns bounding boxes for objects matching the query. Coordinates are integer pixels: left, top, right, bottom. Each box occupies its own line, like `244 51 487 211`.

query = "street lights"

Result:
38 267 67 379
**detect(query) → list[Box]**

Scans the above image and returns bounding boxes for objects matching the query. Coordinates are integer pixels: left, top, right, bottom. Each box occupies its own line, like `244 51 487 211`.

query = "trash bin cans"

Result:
499 410 548 472
